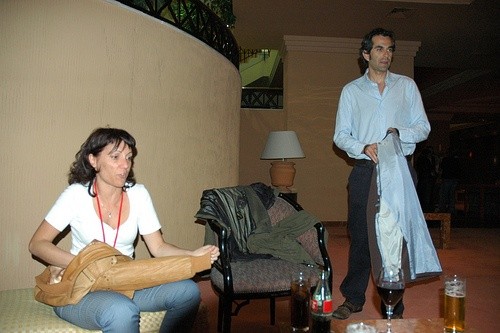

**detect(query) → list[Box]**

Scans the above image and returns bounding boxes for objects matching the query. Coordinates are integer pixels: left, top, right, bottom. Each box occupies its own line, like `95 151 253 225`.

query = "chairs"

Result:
202 182 332 333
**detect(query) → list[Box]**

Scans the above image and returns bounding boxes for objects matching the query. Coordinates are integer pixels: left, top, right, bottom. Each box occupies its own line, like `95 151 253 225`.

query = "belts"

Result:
354 155 413 168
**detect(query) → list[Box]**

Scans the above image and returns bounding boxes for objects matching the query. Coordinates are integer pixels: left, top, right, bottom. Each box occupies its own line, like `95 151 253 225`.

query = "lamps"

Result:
261 130 306 194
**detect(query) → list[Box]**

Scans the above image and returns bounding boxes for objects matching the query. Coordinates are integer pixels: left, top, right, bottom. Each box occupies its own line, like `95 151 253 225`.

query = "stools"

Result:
424 213 451 250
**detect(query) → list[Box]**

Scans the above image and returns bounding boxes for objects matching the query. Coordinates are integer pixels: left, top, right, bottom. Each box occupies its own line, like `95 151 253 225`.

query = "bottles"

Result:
310 270 333 333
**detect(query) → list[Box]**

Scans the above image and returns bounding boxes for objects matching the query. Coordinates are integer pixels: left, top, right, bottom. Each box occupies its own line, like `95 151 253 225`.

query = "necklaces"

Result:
94 181 123 249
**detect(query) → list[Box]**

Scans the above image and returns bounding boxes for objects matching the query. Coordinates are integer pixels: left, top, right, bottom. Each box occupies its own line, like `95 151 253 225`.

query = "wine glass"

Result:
377 266 405 333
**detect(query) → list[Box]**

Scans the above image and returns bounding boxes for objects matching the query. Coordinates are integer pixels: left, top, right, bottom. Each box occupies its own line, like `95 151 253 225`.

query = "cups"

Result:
443 274 467 333
290 271 311 332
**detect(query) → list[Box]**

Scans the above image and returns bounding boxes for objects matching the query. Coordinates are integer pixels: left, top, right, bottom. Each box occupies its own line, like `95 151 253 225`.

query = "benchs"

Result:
0 287 207 333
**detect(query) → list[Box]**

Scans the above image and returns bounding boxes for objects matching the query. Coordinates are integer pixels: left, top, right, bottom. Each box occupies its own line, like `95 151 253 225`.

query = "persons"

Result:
331 28 444 320
28 128 220 333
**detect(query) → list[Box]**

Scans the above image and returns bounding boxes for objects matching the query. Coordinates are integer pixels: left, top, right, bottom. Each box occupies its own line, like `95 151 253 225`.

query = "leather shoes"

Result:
332 298 363 319
381 297 404 319
